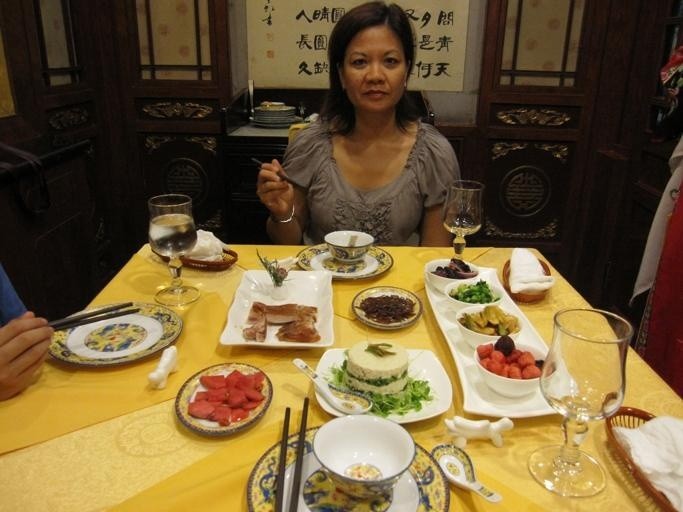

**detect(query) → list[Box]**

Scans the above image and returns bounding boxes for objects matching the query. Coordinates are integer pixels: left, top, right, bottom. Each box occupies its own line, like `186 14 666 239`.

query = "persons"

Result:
0 264 54 402
256 0 462 247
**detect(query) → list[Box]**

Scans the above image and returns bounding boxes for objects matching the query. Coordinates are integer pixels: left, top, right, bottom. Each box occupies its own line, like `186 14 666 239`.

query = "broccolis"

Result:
450 278 500 304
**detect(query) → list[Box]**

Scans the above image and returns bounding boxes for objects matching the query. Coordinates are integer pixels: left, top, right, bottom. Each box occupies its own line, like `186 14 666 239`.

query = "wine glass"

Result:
442 180 485 260
527 305 635 499
147 192 201 310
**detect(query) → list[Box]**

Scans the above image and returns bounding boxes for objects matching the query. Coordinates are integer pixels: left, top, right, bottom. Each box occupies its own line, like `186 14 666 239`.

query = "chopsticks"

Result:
43 302 142 332
275 396 309 511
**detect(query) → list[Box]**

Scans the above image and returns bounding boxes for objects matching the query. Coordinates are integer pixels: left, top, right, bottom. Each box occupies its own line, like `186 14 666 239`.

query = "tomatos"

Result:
476 335 551 380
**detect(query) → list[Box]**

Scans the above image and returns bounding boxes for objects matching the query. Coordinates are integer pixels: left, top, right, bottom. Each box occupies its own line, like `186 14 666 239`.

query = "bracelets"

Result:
270 204 294 224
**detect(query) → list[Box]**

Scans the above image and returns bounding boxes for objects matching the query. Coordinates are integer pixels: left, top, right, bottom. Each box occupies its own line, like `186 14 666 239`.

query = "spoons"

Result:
430 444 503 504
292 356 373 415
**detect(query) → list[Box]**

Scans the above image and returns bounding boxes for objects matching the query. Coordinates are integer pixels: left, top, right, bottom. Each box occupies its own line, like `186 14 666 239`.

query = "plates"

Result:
244 423 449 512
351 284 423 331
219 270 335 349
175 361 273 438
250 102 303 129
46 300 184 368
296 242 394 281
313 346 454 423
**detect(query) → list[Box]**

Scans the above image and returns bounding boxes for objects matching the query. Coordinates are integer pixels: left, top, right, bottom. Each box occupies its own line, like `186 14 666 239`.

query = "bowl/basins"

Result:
444 280 505 316
455 306 525 350
473 340 558 398
312 414 417 485
422 258 483 294
323 230 375 265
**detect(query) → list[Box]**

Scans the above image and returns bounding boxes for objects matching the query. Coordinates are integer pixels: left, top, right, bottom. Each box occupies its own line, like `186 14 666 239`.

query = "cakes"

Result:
344 340 408 396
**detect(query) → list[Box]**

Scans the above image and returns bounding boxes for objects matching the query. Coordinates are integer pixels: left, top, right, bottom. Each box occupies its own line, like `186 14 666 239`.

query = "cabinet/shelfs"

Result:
217 85 312 243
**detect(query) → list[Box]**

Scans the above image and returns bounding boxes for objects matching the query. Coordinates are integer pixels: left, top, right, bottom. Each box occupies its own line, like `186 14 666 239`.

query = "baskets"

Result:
606 406 681 512
155 246 238 272
502 259 551 302
